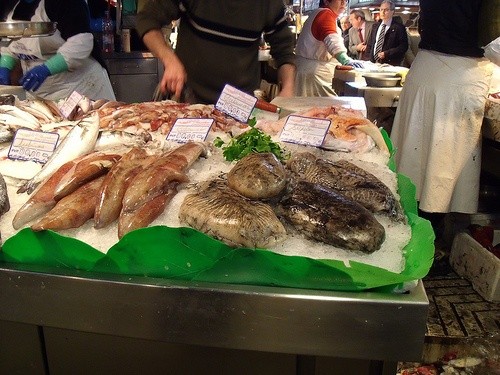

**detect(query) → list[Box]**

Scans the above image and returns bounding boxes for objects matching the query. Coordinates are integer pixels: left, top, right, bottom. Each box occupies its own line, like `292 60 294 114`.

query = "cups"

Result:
121 29 130 53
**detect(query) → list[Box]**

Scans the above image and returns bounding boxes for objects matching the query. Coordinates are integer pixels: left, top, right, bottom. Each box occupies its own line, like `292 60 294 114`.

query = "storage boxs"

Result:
449 230 500 303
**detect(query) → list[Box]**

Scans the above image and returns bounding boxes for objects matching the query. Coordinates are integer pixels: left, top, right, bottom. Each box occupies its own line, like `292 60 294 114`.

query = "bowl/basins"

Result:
362 73 402 87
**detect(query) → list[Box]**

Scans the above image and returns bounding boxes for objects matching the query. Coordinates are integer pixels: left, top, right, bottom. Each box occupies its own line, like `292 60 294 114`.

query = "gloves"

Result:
336 52 364 69
0 54 19 85
19 53 68 93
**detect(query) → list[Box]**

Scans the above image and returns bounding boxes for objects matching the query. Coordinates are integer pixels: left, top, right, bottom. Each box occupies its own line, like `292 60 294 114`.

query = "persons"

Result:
362 0 409 66
348 10 379 60
134 0 295 105
389 0 500 243
0 0 117 103
294 0 362 97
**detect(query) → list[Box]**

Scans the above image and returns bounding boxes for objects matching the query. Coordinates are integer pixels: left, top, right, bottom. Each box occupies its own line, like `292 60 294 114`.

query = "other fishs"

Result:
0 86 408 254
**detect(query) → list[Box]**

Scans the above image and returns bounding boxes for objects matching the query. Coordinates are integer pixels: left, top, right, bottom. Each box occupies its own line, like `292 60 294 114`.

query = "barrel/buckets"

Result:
470 213 491 226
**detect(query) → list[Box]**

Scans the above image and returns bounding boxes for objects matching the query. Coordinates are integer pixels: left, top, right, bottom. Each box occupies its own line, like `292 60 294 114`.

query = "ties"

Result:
373 24 386 61
357 28 365 60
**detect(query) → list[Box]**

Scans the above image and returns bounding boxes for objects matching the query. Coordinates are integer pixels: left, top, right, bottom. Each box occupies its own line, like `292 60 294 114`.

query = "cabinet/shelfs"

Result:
103 52 166 102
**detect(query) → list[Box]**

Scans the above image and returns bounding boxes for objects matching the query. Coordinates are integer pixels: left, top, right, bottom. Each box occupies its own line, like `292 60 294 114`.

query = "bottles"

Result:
102 10 114 55
